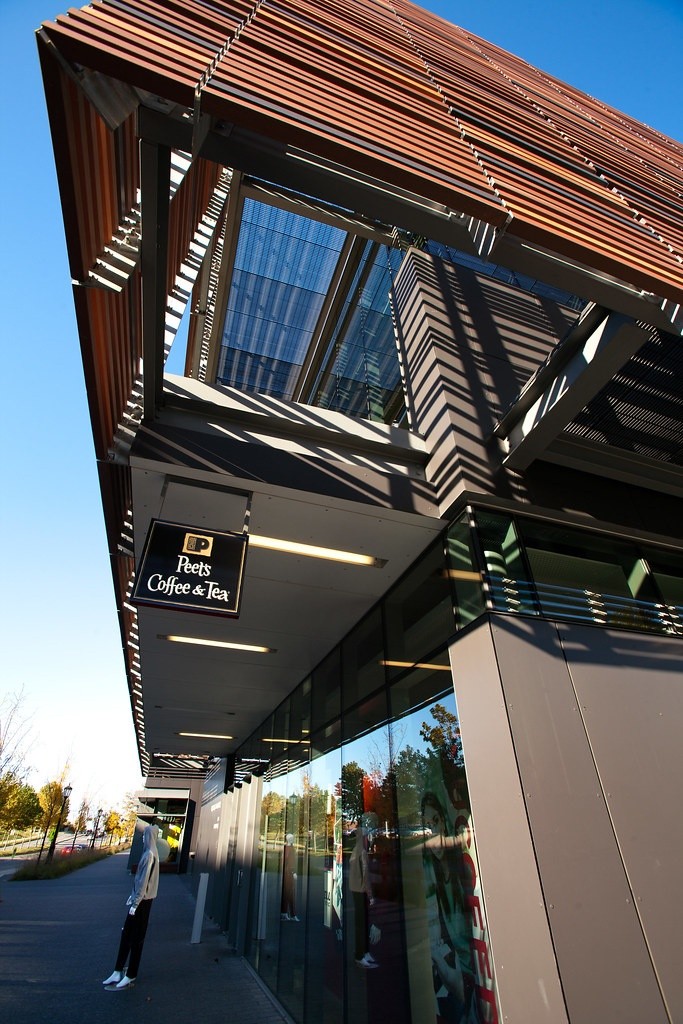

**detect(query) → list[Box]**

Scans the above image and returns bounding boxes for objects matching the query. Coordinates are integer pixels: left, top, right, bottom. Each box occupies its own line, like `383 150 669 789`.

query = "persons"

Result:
103 826 159 987
349 813 386 969
281 834 300 920
422 795 483 1024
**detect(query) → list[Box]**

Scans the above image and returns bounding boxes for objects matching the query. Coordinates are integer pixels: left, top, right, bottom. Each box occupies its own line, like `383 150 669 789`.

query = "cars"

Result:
343 825 433 838
60 844 96 855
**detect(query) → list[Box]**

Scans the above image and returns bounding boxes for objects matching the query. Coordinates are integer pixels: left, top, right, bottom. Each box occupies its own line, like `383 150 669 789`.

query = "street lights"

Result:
91 807 103 850
45 782 72 865
